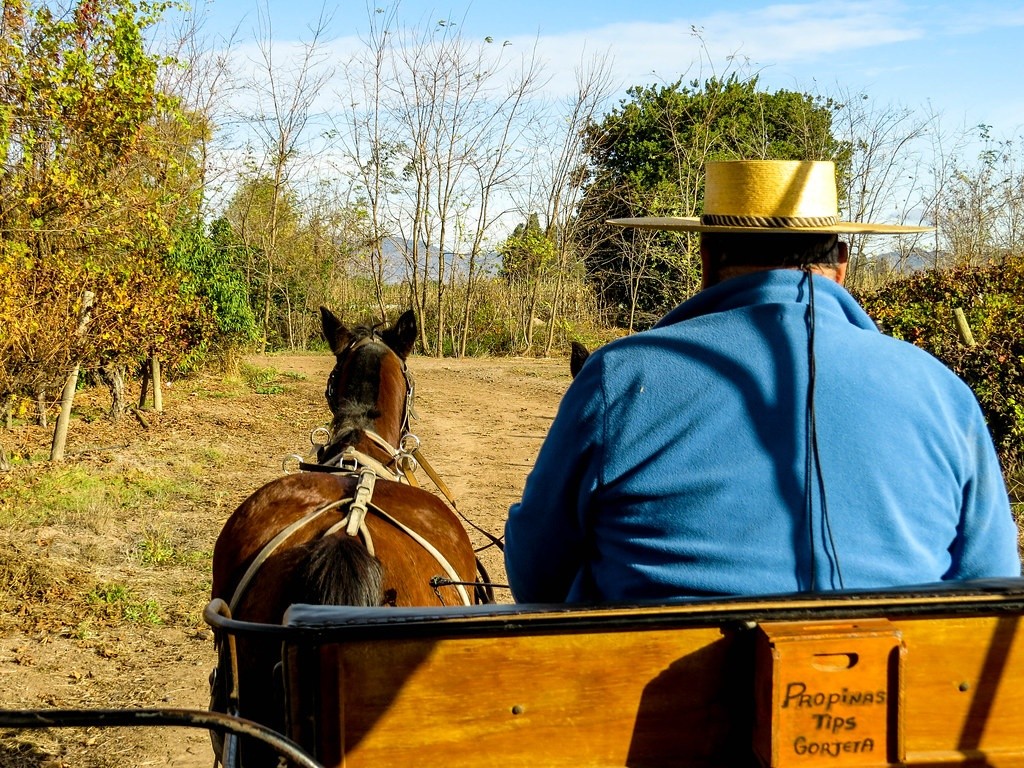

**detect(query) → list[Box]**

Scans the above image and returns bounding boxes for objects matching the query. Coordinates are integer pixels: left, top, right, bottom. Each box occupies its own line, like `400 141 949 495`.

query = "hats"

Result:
606 159 938 233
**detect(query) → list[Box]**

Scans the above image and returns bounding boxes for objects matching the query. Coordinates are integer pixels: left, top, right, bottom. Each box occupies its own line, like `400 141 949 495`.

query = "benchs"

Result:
203 577 1024 768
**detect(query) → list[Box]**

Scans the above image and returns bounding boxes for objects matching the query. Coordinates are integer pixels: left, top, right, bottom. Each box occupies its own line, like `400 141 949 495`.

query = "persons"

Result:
504 160 1022 606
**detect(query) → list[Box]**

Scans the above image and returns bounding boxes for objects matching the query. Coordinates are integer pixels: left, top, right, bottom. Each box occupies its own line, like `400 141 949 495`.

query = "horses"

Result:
204 305 589 768
